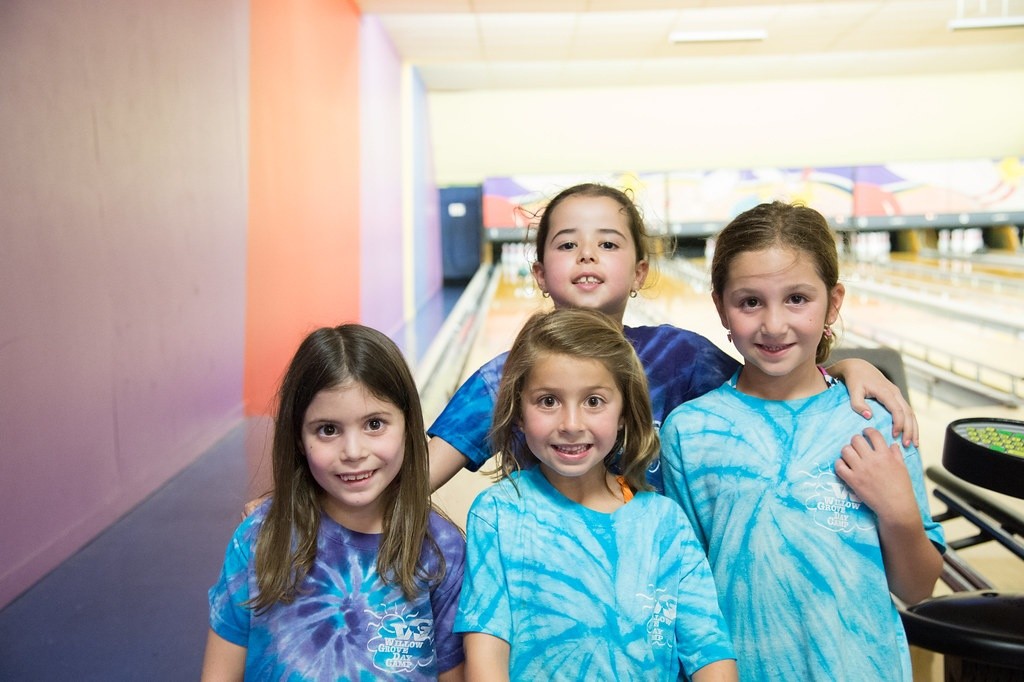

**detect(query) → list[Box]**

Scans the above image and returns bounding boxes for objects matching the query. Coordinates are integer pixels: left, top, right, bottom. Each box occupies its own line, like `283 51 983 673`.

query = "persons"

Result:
242 184 920 493
453 308 738 682
201 323 469 682
660 201 947 682
